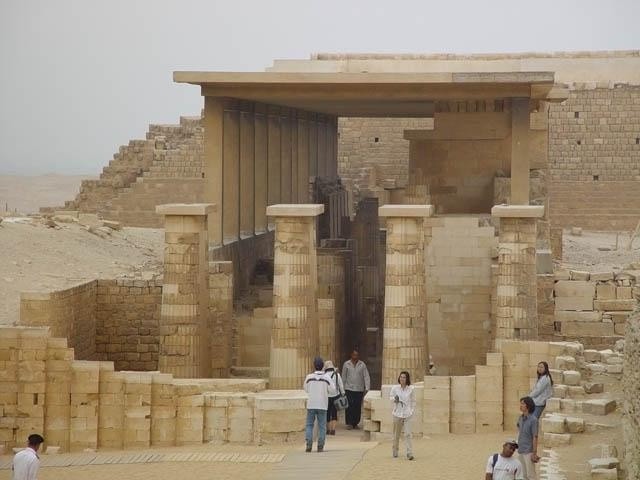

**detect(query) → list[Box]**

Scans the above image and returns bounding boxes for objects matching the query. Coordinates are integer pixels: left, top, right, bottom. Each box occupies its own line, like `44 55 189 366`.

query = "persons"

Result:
486 362 553 480
303 350 417 461
13 434 44 480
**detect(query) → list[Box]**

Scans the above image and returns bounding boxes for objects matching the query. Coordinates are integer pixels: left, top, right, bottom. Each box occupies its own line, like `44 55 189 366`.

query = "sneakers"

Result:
306 448 313 452
347 425 359 430
318 448 323 452
326 429 337 435
407 455 414 460
393 451 398 457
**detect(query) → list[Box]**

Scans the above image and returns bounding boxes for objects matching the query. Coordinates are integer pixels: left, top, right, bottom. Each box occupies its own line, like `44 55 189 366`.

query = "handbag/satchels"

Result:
334 396 349 410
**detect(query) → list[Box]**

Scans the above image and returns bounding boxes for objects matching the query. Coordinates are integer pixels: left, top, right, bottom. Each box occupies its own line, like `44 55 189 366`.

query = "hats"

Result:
314 357 322 368
28 434 43 442
323 360 334 370
